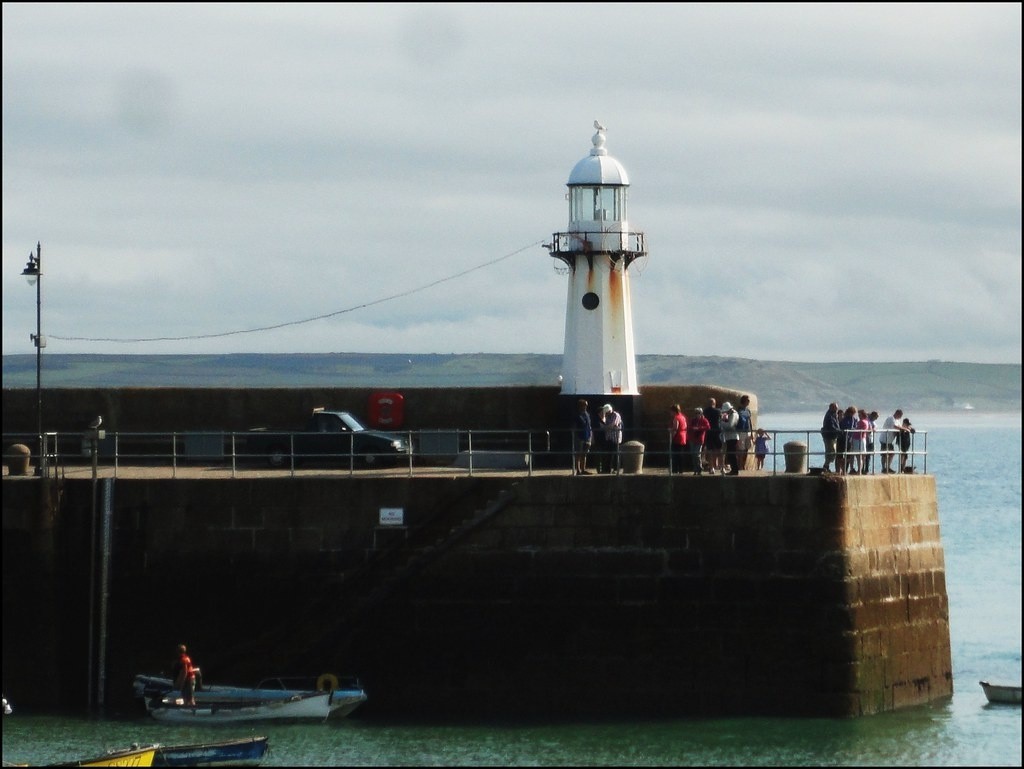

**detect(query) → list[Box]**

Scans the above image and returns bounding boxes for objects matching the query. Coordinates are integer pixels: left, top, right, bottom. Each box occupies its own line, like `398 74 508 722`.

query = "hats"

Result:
603 404 612 412
903 418 912 425
722 402 734 412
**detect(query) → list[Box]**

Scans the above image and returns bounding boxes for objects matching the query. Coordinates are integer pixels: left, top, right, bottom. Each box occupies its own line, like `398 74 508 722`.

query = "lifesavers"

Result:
316 673 338 693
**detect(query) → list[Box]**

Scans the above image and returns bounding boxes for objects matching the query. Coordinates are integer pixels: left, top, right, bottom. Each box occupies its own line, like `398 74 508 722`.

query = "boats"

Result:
2 734 272 767
134 665 374 730
978 677 1021 704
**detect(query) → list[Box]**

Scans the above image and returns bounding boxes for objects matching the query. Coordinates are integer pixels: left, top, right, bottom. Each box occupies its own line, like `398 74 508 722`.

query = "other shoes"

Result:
725 470 739 476
882 467 896 473
720 468 725 473
864 470 871 474
709 467 715 474
849 469 857 475
576 471 594 475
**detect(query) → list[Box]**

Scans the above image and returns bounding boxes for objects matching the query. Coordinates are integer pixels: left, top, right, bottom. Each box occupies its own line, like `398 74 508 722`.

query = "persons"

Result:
820 402 842 469
850 408 869 474
687 406 711 473
174 645 199 708
572 400 594 476
894 417 915 472
753 427 772 470
733 395 754 470
879 409 910 475
716 401 741 476
600 405 623 475
669 403 687 474
837 404 860 474
702 396 725 474
865 410 879 474
835 408 846 431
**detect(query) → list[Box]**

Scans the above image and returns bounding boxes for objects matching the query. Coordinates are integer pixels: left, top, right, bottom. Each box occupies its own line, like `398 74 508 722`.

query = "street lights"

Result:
24 239 49 478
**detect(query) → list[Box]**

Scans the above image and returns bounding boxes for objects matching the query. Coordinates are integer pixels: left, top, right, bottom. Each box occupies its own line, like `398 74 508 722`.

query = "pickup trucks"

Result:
246 407 414 470
